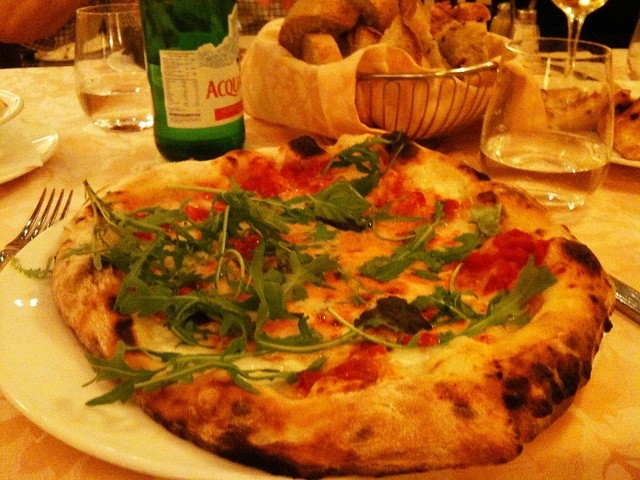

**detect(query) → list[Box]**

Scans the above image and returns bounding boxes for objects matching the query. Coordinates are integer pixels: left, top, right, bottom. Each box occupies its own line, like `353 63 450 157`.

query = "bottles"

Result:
488 3 512 36
513 11 540 52
138 1 247 162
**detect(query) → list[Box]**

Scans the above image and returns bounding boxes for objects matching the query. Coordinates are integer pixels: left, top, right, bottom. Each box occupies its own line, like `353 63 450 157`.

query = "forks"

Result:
1 188 73 273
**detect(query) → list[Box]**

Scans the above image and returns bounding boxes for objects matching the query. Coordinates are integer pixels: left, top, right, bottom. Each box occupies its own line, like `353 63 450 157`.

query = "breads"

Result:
278 0 504 74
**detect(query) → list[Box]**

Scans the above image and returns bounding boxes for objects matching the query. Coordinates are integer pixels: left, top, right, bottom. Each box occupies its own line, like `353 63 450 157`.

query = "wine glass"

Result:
552 1 605 74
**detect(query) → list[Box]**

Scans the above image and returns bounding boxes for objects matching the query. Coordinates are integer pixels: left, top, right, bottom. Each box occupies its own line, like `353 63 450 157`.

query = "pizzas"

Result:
52 134 617 472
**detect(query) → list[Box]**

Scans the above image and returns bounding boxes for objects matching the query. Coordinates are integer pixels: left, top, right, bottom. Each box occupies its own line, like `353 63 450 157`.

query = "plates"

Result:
1 215 294 480
1 100 57 186
610 81 640 168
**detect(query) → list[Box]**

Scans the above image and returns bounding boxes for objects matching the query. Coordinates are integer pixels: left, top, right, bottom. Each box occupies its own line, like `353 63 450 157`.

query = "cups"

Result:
1 91 24 124
628 22 640 79
477 38 617 206
75 5 155 134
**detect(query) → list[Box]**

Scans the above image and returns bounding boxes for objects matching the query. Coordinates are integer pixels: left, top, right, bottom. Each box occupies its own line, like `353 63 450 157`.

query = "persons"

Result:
0 1 285 66
